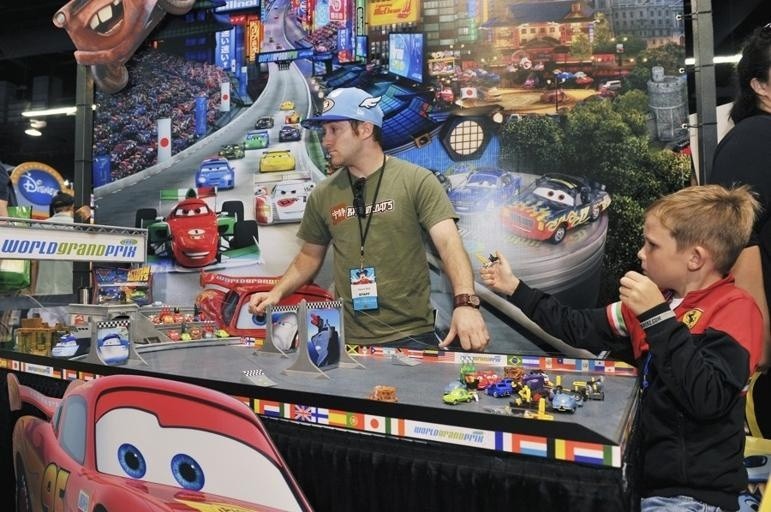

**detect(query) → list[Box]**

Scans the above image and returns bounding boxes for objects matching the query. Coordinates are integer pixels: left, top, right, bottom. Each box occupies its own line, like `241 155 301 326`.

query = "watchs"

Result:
453 293 479 309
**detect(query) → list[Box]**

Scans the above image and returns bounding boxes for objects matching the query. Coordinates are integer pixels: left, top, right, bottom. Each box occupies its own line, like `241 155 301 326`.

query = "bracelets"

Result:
639 310 676 329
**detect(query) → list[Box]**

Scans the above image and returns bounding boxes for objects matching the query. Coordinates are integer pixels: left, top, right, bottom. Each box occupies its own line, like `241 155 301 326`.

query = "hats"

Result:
300 87 384 132
51 193 73 208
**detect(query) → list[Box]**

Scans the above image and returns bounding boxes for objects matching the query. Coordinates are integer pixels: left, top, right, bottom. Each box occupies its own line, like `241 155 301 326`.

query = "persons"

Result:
247 86 490 352
705 21 771 507
478 185 761 512
0 166 12 225
351 269 376 284
325 319 331 326
